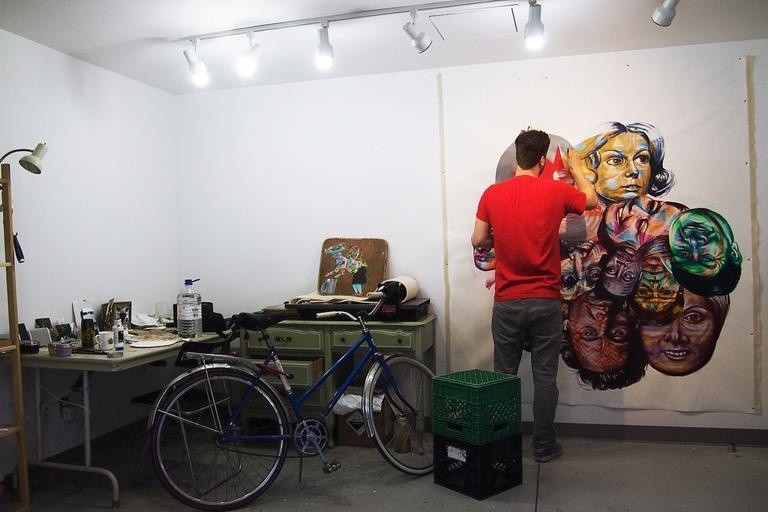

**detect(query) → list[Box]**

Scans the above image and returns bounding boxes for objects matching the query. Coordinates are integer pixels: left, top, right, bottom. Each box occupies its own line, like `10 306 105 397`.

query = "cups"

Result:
95 331 114 350
47 342 72 356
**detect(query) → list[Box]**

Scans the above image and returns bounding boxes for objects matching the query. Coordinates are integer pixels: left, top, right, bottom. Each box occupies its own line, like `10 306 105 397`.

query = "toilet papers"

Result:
382 276 419 302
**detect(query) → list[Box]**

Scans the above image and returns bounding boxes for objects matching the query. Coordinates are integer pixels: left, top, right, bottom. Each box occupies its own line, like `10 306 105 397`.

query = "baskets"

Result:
431 368 522 445
433 422 522 501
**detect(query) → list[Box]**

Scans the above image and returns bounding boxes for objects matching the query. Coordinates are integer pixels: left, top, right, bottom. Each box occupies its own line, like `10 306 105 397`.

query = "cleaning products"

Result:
177 278 203 337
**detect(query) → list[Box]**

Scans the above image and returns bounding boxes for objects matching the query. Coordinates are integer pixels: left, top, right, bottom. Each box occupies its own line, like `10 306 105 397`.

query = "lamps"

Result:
0 141 49 176
179 0 681 96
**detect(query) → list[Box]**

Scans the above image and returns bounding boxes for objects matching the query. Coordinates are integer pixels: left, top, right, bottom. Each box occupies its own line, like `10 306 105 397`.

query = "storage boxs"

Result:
333 385 394 448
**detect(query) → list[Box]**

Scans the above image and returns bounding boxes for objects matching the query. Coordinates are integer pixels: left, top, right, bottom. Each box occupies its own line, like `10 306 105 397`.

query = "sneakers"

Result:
534 443 563 462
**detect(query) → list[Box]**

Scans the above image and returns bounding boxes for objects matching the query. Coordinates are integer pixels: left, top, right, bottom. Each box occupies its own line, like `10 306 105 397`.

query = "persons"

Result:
473 227 496 290
324 242 368 297
472 130 599 462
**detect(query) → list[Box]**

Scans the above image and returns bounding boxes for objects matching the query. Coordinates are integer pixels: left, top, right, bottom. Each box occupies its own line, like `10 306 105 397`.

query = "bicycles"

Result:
146 292 436 511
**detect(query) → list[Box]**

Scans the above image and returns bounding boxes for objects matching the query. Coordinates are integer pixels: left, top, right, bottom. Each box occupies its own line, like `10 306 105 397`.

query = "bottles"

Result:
80 318 95 347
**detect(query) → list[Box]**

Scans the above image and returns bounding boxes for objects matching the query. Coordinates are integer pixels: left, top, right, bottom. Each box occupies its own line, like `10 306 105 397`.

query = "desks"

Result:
0 328 239 512
240 314 435 450
